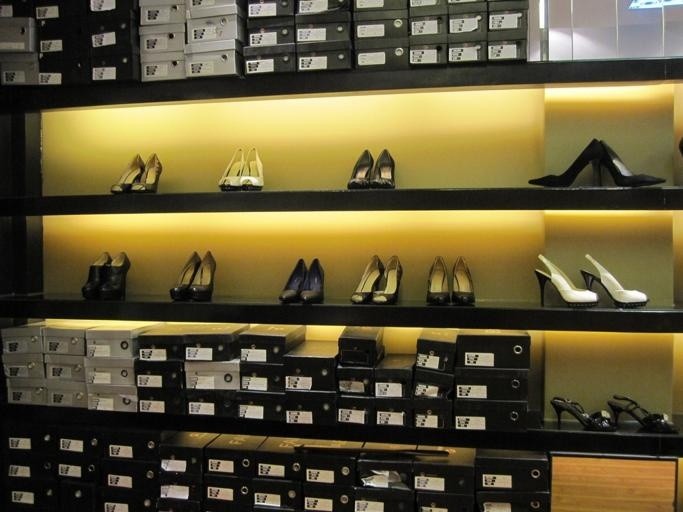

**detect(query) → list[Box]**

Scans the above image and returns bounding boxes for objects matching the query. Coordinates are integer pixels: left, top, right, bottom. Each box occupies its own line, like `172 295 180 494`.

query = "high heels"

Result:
277 254 326 306
607 393 677 434
581 249 651 310
598 140 667 188
217 145 265 193
549 396 617 432
168 250 218 303
346 147 396 192
451 255 476 306
80 251 131 297
528 137 600 188
535 252 600 308
424 254 450 307
110 152 163 198
350 254 404 307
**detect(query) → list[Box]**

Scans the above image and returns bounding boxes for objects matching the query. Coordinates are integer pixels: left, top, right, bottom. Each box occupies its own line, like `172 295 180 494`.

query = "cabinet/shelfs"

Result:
0 57 683 454
